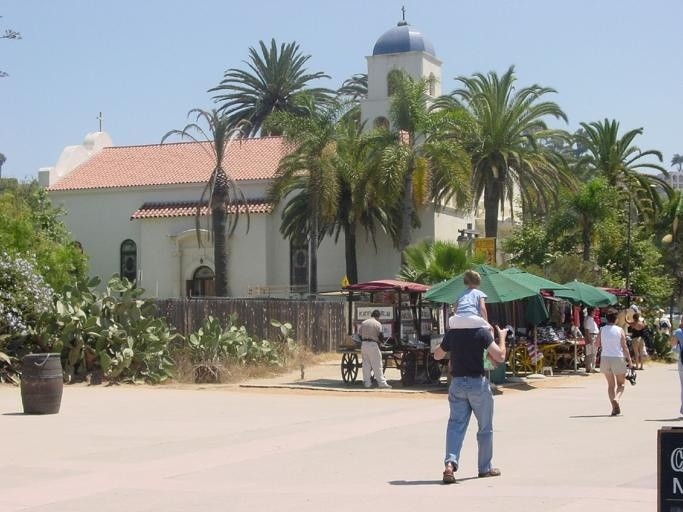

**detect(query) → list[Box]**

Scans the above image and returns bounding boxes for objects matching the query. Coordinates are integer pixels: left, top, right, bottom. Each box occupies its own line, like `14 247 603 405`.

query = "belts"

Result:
361 338 377 342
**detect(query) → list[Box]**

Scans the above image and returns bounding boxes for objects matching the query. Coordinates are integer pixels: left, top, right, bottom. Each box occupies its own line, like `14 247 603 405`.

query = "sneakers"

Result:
611 399 620 415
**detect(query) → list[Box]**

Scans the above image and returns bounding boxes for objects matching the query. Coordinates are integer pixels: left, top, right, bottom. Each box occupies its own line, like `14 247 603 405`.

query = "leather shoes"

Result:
379 385 392 389
478 469 500 477
442 469 457 483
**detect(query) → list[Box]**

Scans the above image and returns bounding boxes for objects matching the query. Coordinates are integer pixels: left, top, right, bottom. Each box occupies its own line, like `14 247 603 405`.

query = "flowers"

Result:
0 251 55 336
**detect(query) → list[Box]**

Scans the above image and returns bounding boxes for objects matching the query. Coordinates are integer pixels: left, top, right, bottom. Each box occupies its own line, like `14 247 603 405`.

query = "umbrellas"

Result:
554 278 618 309
499 266 576 373
418 262 542 307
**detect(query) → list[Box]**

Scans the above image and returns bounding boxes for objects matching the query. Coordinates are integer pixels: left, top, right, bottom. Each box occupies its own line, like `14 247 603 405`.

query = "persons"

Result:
430 324 510 481
447 269 494 343
671 314 683 415
359 310 388 389
594 307 632 418
654 309 670 335
626 311 648 372
582 305 599 372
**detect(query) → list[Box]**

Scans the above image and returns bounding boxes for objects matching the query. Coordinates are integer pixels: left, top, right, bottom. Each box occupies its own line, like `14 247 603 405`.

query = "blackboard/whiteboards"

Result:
657 429 683 512
